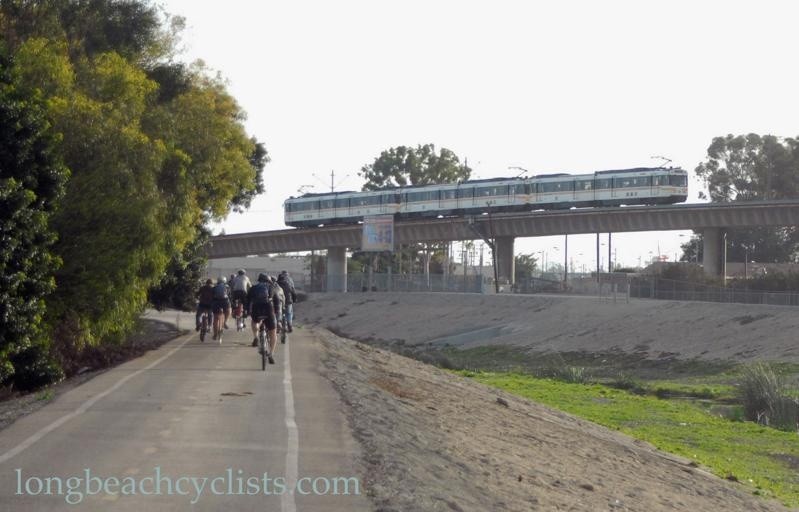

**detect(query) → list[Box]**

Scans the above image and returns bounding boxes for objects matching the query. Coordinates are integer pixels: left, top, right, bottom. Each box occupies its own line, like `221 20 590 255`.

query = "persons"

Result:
194 269 296 365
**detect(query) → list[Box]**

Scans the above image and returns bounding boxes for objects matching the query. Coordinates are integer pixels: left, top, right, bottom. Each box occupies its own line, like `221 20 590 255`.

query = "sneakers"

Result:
238 322 247 332
268 353 275 365
277 320 281 329
252 338 259 347
224 321 229 330
207 326 218 342
195 324 200 332
288 320 293 332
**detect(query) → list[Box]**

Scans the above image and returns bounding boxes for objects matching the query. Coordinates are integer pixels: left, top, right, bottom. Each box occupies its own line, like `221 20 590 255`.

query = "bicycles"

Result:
199 293 288 371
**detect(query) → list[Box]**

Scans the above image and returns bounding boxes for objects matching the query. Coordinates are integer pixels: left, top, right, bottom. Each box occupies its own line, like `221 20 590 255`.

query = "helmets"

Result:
257 273 272 282
217 275 227 284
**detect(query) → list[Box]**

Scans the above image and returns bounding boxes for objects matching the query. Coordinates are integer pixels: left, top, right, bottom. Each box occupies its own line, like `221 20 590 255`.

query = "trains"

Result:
284 156 688 228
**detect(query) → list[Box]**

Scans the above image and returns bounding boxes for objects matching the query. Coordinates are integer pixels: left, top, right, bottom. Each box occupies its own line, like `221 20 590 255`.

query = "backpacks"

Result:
214 285 228 300
200 286 214 307
254 283 273 305
